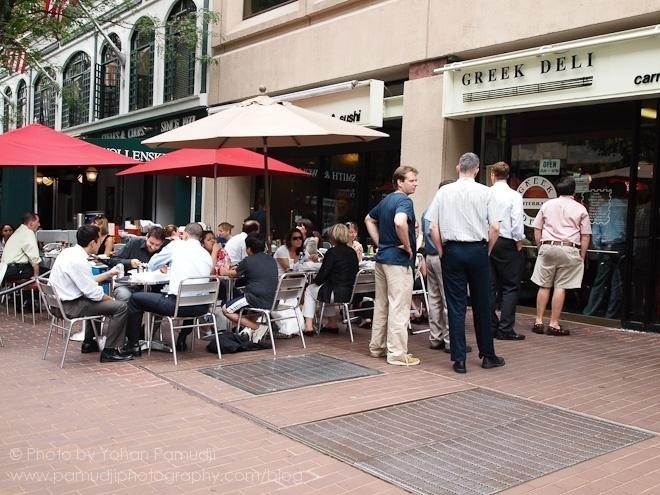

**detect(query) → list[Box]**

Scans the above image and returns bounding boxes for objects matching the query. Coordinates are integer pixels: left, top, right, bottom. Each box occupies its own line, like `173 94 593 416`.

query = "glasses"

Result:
291 237 303 241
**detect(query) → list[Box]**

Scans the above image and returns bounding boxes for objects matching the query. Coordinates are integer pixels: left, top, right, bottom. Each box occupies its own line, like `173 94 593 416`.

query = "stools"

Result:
2 276 53 325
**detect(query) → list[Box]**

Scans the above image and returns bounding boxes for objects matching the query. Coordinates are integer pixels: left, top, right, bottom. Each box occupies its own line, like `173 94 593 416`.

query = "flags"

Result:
43 0 77 40
1 48 25 74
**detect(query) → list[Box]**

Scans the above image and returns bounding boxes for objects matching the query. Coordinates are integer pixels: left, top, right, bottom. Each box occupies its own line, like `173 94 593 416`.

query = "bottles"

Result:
298 251 304 265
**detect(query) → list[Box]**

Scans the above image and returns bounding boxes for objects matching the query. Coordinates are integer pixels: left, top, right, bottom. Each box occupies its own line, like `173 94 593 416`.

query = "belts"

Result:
8 263 22 266
541 240 580 249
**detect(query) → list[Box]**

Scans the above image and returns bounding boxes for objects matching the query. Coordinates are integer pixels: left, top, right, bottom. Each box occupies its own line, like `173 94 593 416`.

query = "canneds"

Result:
115 262 125 279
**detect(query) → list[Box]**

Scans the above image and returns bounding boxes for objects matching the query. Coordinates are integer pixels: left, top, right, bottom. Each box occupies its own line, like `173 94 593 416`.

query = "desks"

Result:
39 255 109 301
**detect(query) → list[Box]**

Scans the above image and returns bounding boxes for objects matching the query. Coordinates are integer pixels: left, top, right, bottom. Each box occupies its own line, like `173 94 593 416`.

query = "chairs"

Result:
31 274 109 368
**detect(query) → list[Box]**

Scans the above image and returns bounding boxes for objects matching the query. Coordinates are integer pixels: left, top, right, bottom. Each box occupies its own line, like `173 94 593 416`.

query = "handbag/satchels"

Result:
206 331 272 354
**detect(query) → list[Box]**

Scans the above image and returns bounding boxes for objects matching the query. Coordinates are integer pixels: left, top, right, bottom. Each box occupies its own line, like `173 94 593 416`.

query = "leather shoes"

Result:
547 324 570 335
82 340 99 352
497 333 525 340
321 327 339 333
532 322 544 333
303 331 313 336
15 295 39 314
453 360 466 372
482 357 505 368
177 339 187 351
100 351 133 362
120 344 141 357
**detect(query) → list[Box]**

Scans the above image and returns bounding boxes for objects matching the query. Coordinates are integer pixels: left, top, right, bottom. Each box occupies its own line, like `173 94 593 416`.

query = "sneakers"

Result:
202 330 222 339
251 323 269 343
430 341 471 353
387 353 420 366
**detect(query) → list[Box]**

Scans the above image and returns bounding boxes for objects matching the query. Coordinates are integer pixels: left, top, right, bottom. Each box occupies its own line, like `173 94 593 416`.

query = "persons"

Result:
488 162 526 340
364 167 421 365
48 210 362 361
0 210 50 313
577 178 652 319
423 152 505 372
530 175 592 335
422 179 471 353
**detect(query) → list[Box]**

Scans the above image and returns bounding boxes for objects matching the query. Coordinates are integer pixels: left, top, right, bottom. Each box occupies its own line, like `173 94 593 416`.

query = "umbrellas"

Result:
0 117 147 215
140 87 391 255
115 147 311 236
